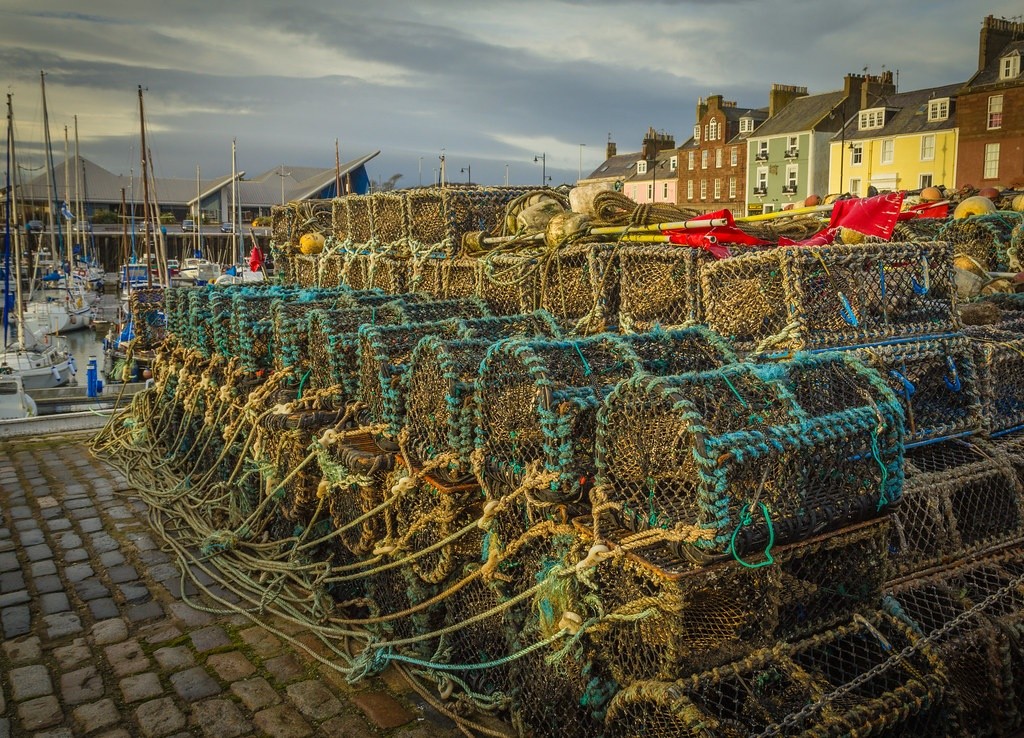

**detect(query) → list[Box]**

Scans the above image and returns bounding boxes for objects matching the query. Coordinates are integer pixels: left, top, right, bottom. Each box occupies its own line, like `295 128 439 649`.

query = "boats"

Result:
1 67 271 431
